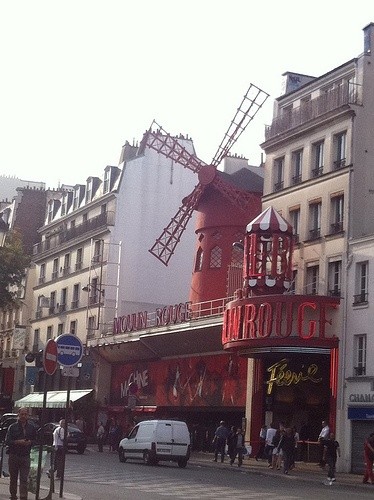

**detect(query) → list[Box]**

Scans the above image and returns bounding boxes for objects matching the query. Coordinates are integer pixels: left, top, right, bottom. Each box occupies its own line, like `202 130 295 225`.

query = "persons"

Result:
227 426 236 456
211 420 230 462
254 424 268 461
268 422 287 470
266 423 277 466
317 419 330 467
292 425 300 468
322 432 341 482
108 420 117 451
231 427 245 467
97 420 105 452
278 426 295 474
45 418 67 480
362 432 374 484
4 407 38 499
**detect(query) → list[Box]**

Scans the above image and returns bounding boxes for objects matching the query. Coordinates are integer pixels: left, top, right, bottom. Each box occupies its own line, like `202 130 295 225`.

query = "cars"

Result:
33 421 88 454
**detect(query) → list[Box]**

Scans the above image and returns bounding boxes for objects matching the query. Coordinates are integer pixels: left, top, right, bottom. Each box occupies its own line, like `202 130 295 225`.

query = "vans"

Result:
117 419 192 469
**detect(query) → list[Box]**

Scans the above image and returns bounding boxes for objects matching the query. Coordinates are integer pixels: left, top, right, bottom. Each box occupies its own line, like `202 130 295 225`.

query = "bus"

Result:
0 412 41 447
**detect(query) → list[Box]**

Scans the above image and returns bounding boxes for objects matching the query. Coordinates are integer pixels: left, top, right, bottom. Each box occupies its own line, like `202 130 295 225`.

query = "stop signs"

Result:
43 338 58 376
